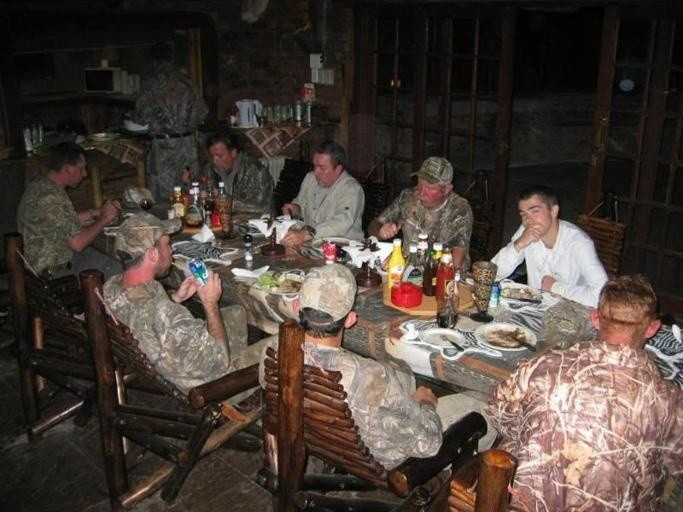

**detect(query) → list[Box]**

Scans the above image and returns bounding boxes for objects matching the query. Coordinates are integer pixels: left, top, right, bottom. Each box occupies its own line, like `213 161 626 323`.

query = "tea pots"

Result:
233 99 261 127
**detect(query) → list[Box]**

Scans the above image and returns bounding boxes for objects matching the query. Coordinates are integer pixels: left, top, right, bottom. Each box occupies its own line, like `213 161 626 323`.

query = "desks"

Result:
38 137 152 209
228 124 332 166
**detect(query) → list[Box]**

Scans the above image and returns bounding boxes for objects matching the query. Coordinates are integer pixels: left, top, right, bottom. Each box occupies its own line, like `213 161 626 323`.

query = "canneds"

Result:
322 240 338 266
489 280 501 309
188 257 208 287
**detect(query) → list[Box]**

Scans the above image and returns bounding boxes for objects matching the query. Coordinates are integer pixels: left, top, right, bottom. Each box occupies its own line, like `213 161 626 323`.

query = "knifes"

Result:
509 333 537 352
504 294 542 305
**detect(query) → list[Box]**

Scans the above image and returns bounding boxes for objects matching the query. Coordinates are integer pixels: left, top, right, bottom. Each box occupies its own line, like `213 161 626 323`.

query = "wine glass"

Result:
140 198 153 213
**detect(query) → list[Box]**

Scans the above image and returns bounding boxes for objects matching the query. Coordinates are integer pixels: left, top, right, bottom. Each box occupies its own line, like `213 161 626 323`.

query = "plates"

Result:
498 276 545 306
250 272 305 295
103 212 136 228
474 323 538 353
89 132 120 141
418 328 466 349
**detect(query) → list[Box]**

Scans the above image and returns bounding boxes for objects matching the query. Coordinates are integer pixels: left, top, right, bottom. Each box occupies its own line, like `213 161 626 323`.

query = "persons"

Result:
258 262 500 494
484 271 683 512
489 185 609 309
367 155 474 273
100 209 271 408
181 133 278 217
280 139 367 249
129 35 210 199
15 141 124 326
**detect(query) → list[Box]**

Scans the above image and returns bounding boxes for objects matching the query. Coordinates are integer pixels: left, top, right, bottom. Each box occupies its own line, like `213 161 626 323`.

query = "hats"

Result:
408 155 455 187
111 209 184 262
297 262 358 328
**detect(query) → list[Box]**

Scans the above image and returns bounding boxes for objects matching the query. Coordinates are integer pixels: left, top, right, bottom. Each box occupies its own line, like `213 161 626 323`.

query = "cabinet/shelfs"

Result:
0 12 219 160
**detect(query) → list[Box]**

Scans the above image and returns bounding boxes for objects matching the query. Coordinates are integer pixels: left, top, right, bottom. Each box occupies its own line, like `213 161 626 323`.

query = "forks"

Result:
439 334 464 353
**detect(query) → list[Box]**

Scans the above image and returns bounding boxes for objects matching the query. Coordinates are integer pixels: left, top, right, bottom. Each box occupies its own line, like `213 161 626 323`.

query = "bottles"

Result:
167 166 238 234
261 100 313 127
384 233 461 309
22 120 44 152
226 111 238 126
242 233 255 261
488 281 500 307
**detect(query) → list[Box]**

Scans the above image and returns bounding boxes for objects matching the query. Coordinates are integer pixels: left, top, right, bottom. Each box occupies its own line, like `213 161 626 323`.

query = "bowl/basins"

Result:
310 236 364 257
